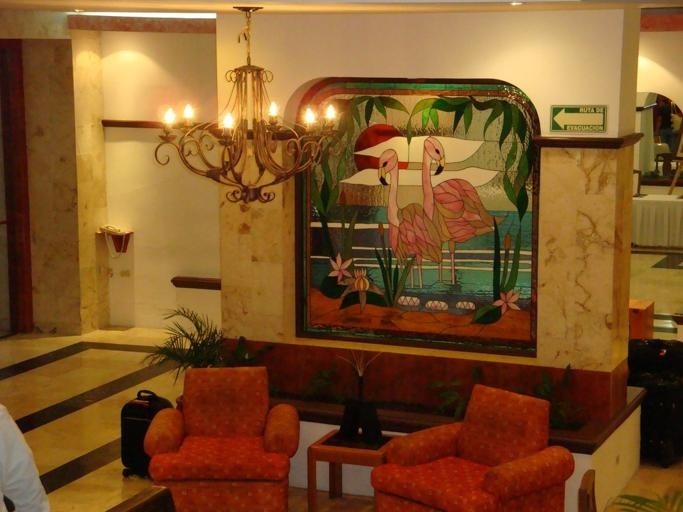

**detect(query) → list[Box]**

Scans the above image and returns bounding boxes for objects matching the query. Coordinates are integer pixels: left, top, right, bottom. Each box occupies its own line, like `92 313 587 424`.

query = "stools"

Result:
304 429 400 509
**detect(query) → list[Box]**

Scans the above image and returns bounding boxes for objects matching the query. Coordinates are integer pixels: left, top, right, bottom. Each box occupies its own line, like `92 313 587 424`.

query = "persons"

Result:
0 404 50 512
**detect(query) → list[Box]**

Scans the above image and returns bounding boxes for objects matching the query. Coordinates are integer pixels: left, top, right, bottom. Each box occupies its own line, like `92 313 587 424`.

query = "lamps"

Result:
153 5 344 211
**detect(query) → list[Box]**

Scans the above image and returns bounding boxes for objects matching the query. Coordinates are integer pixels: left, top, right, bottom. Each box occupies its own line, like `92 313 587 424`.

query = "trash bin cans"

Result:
628 338 682 467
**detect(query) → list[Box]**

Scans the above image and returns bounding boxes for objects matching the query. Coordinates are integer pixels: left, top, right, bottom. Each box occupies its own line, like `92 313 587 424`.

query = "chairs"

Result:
370 382 574 512
578 464 598 511
103 483 176 511
143 364 301 510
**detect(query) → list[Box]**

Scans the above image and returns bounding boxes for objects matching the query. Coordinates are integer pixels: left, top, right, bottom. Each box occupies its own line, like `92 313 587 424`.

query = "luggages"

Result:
114 385 175 483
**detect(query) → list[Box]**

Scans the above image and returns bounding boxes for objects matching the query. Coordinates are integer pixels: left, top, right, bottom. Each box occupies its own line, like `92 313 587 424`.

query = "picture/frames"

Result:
291 75 540 360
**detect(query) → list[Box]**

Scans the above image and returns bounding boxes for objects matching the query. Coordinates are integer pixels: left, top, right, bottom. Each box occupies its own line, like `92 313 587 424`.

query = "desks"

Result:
628 298 656 338
630 191 682 251
